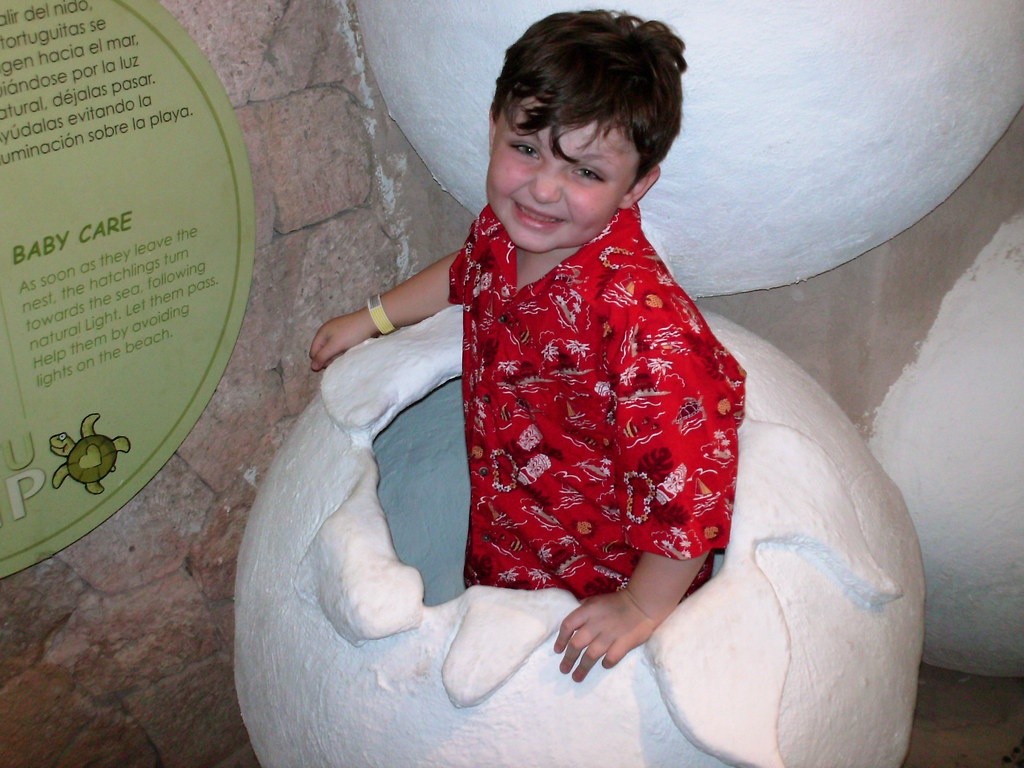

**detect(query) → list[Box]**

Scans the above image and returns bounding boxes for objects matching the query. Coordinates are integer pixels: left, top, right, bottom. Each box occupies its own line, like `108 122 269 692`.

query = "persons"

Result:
310 9 746 684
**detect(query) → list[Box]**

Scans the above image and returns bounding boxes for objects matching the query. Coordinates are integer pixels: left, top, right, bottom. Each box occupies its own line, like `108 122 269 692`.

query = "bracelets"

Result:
367 293 395 335
